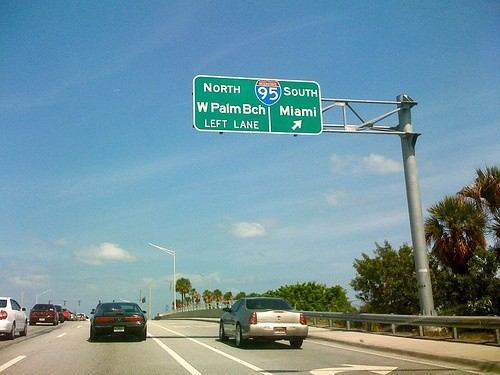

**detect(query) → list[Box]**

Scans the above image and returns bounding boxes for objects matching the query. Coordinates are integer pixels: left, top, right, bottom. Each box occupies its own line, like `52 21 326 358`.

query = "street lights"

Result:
35 288 53 305
148 242 177 312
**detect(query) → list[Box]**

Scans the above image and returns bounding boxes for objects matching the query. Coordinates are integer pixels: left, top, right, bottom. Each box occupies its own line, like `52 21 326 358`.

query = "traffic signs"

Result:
191 74 324 136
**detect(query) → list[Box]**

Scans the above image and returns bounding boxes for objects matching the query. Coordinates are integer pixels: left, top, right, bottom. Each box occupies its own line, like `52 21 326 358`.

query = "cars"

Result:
0 296 28 339
218 296 309 349
55 305 86 323
88 302 148 341
30 304 59 326
